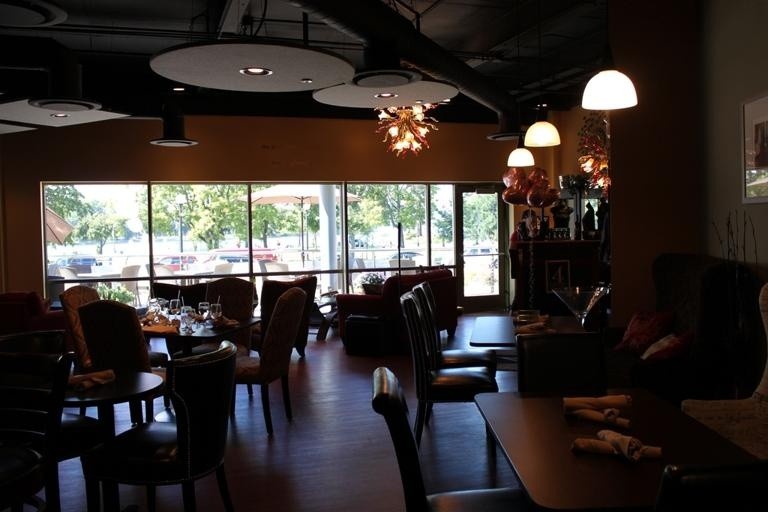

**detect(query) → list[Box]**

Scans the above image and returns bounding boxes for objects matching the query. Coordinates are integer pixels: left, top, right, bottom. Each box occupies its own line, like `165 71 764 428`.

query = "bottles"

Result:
547 227 570 240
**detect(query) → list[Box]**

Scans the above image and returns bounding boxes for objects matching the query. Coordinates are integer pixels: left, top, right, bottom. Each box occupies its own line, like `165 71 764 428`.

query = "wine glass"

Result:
169 298 223 334
551 283 613 329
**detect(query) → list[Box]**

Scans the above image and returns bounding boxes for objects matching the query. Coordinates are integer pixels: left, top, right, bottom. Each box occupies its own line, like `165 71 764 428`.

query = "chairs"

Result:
151 277 262 399
371 367 536 511
2 277 169 510
92 340 238 510
310 266 458 363
232 285 307 434
412 281 497 426
399 290 500 454
252 276 317 359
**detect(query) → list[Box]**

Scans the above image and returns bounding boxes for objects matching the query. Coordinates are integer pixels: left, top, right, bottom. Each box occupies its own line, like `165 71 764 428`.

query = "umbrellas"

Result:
45 205 74 245
237 183 360 268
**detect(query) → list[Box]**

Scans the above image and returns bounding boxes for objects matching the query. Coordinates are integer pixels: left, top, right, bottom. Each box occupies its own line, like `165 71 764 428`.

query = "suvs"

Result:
54 256 99 268
157 255 199 271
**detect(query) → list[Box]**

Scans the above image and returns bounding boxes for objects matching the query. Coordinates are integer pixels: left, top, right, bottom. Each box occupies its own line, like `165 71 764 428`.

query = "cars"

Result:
383 251 424 260
463 246 499 253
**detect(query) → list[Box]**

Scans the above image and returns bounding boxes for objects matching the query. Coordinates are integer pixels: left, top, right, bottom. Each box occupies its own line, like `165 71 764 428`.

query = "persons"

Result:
582 202 595 232
596 197 609 231
509 209 541 317
550 199 573 230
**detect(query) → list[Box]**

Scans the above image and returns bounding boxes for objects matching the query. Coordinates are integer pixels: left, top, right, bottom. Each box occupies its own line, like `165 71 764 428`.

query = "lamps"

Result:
506 140 536 169
580 50 642 111
523 108 562 149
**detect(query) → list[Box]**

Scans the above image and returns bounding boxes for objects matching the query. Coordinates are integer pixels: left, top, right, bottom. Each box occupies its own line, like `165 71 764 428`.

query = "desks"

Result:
474 386 767 510
469 316 600 391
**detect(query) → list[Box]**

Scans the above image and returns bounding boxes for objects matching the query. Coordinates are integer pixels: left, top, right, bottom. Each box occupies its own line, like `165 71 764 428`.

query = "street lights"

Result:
304 203 311 262
174 193 190 286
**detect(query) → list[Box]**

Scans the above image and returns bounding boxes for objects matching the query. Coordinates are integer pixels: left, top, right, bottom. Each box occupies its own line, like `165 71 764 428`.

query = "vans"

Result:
199 247 281 267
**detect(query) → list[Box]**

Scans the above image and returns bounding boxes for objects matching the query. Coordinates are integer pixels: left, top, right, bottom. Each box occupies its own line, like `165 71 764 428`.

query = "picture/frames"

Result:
737 90 768 208
